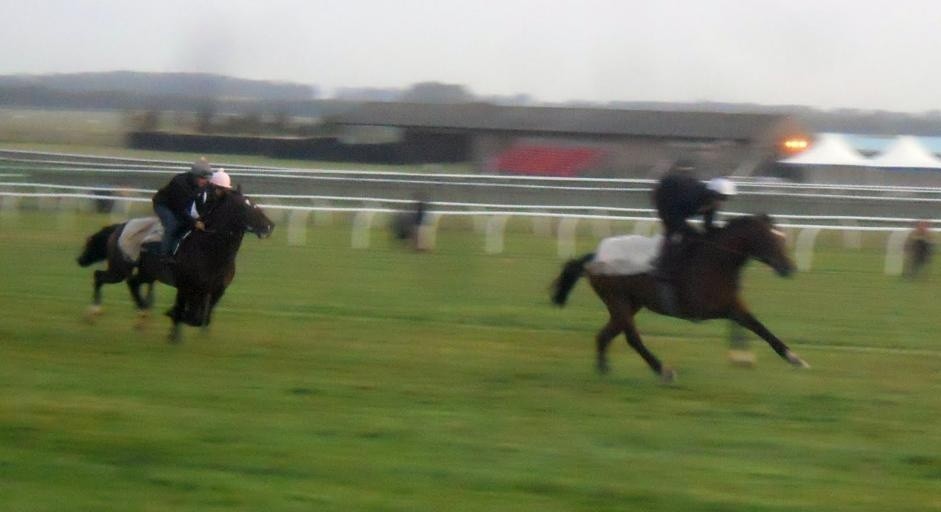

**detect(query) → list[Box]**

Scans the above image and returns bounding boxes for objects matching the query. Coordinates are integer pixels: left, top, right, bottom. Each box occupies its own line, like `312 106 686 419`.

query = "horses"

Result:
554 212 814 385
76 182 274 344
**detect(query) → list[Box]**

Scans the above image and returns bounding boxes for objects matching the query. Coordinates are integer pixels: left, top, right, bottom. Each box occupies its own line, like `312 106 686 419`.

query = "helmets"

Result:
705 176 737 196
190 156 214 179
209 168 233 189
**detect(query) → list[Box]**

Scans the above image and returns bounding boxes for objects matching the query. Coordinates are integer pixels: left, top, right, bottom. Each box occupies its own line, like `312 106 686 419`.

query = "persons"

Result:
650 169 738 271
191 167 232 222
153 157 213 263
